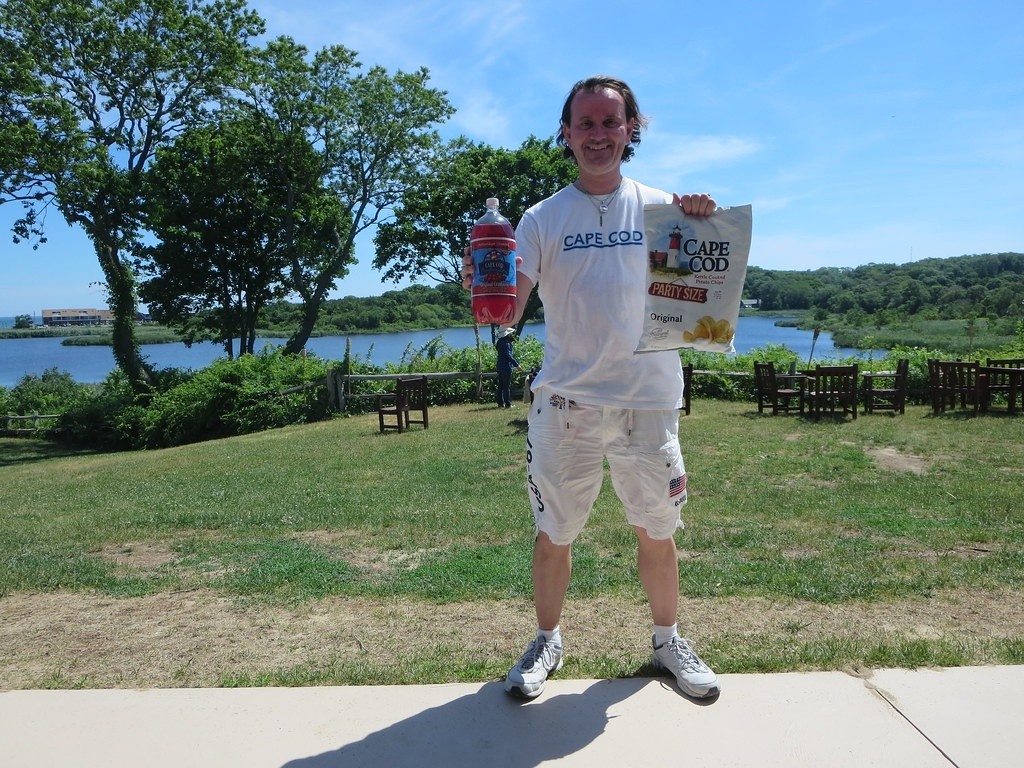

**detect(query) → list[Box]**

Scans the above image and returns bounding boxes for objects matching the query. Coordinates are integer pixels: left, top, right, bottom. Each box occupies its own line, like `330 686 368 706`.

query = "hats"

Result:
497 325 516 338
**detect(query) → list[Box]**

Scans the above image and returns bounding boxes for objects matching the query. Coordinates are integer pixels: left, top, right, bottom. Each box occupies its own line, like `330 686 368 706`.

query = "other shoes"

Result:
498 404 505 408
506 405 515 408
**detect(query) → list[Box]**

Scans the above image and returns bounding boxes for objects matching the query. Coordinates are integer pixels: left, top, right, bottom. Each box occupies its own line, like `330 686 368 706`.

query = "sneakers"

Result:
504 635 563 698
651 633 722 700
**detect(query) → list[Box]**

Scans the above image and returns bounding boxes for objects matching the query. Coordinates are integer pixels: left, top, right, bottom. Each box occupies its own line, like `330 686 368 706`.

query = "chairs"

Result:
379 375 429 434
678 363 693 415
754 361 806 416
807 362 859 422
861 359 909 415
927 358 1023 414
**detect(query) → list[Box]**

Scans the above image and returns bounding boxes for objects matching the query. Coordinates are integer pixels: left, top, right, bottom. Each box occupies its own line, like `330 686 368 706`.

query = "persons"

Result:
461 78 723 701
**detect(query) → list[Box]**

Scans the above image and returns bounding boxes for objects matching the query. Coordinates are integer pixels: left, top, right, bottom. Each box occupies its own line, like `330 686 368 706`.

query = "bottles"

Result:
469 198 517 319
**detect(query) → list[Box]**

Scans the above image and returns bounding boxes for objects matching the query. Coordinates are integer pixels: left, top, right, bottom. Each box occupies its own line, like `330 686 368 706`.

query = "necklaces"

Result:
574 175 624 228
